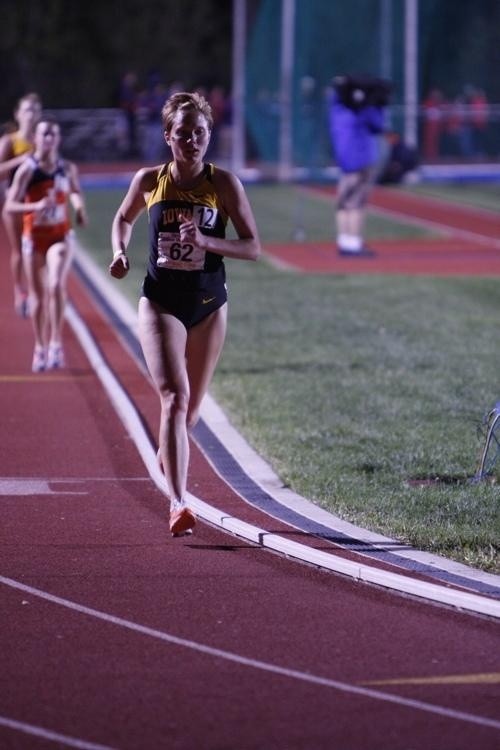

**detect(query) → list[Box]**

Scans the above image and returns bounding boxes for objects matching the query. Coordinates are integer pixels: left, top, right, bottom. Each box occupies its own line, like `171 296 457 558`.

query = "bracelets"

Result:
113 250 123 259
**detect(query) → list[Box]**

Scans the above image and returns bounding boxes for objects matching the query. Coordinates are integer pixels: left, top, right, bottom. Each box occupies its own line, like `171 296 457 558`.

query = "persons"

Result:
469 89 489 154
385 129 418 183
4 114 88 373
327 73 382 256
447 99 466 156
109 90 263 538
117 72 233 160
421 86 445 158
0 88 45 320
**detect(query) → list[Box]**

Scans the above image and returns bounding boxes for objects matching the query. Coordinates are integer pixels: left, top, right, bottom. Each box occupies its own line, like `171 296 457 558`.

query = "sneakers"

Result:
169 504 196 537
31 340 64 372
